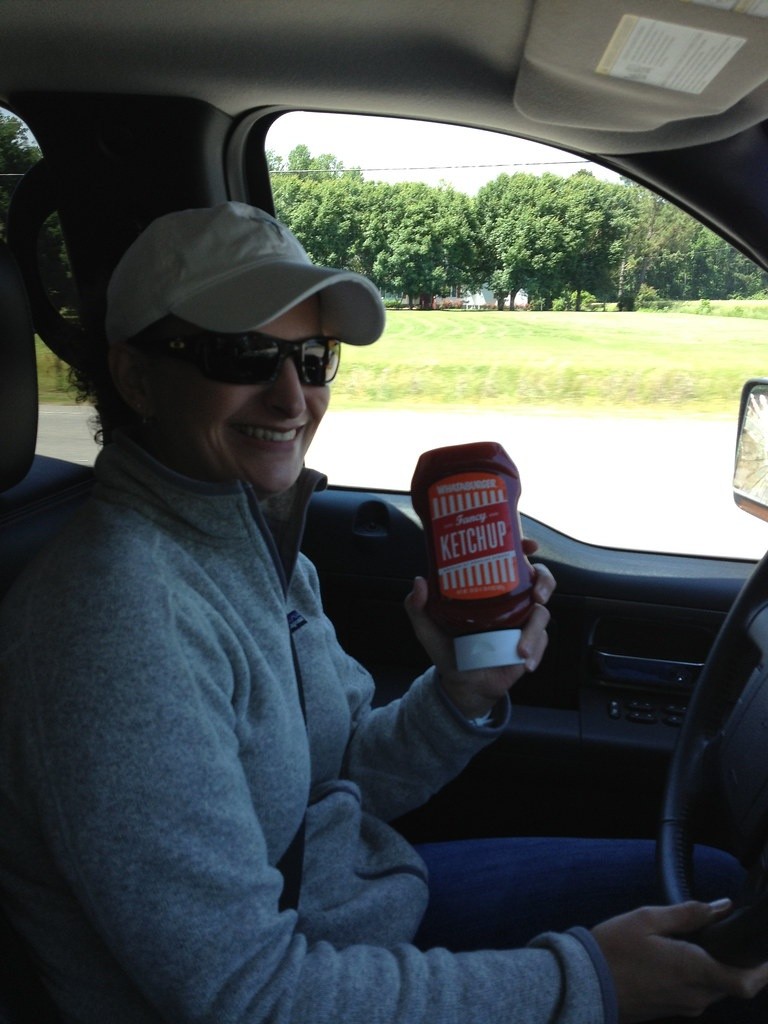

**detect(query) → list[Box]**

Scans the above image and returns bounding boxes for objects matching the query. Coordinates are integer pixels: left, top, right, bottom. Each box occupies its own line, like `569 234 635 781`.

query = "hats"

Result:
105 200 387 348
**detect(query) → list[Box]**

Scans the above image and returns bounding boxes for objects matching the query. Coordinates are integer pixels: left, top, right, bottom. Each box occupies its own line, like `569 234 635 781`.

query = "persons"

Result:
0 199 768 1024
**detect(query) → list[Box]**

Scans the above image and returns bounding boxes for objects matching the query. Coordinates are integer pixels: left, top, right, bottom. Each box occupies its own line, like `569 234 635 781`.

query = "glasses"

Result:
134 324 342 386
